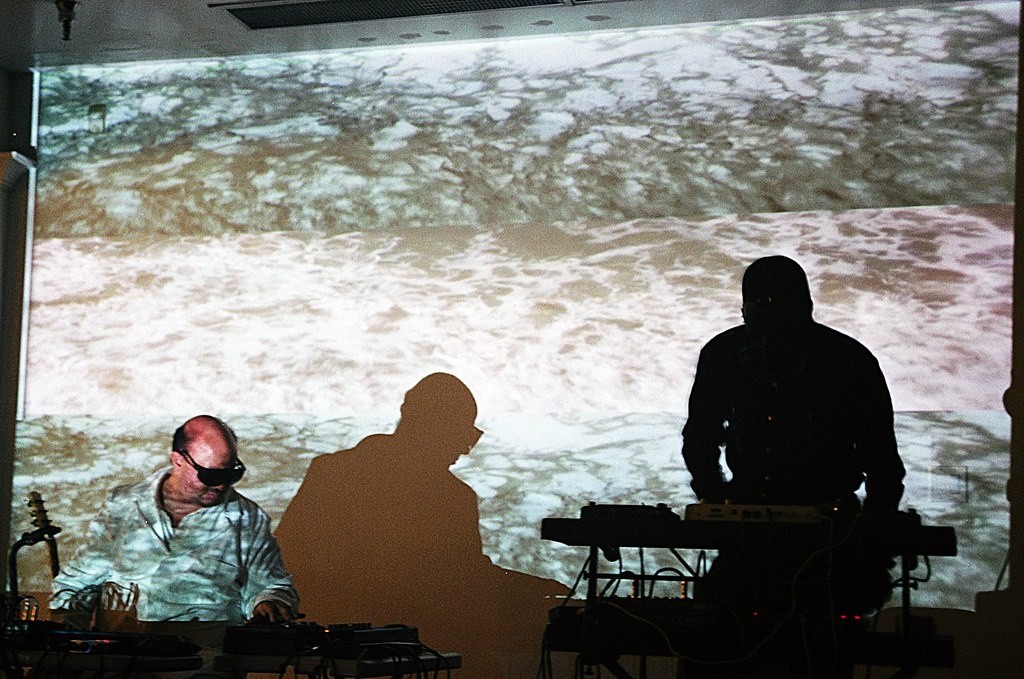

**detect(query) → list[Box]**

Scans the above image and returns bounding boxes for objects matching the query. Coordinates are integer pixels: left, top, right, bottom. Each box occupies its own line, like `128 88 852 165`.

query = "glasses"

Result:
180 449 246 486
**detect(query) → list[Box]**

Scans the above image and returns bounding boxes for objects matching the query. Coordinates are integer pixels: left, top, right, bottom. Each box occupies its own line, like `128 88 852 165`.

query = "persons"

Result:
26 415 300 679
675 254 907 679
